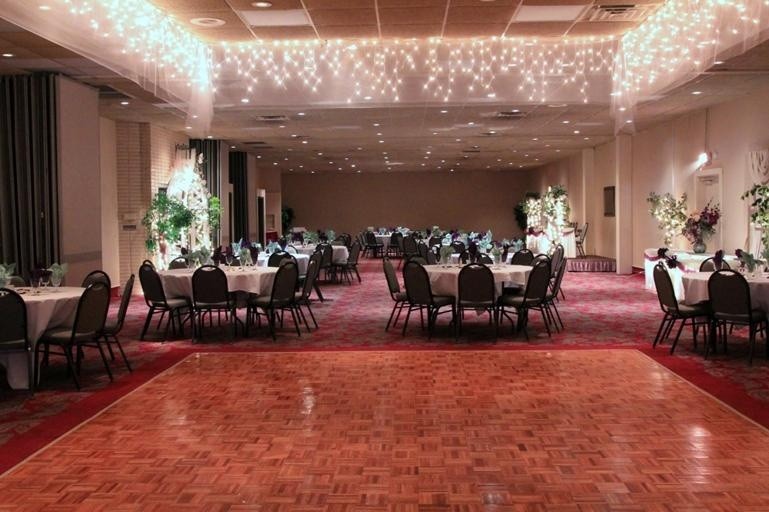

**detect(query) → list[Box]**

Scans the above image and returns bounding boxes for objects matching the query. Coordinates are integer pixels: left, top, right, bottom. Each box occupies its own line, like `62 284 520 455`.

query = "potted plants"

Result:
682 197 723 243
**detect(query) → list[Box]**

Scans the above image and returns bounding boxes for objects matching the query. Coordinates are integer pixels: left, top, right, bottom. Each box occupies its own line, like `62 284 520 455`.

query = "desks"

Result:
643 248 738 304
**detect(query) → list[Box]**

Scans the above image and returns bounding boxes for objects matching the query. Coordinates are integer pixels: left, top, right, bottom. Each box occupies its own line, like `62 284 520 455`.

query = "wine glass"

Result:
435 256 442 268
252 261 258 270
239 258 247 272
30 279 40 296
40 276 51 291
0 281 9 297
190 259 196 273
184 257 190 271
226 256 234 268
442 256 449 270
246 258 252 268
51 277 62 292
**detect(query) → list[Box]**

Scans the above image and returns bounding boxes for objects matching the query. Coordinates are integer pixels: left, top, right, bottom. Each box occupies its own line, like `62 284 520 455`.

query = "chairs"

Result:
652 256 769 366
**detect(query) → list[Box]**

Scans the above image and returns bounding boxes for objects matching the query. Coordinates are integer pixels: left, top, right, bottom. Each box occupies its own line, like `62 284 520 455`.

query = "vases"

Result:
693 239 705 254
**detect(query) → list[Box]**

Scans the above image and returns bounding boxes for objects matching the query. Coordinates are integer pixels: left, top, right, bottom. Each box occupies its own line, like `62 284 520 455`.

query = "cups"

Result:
220 264 224 268
